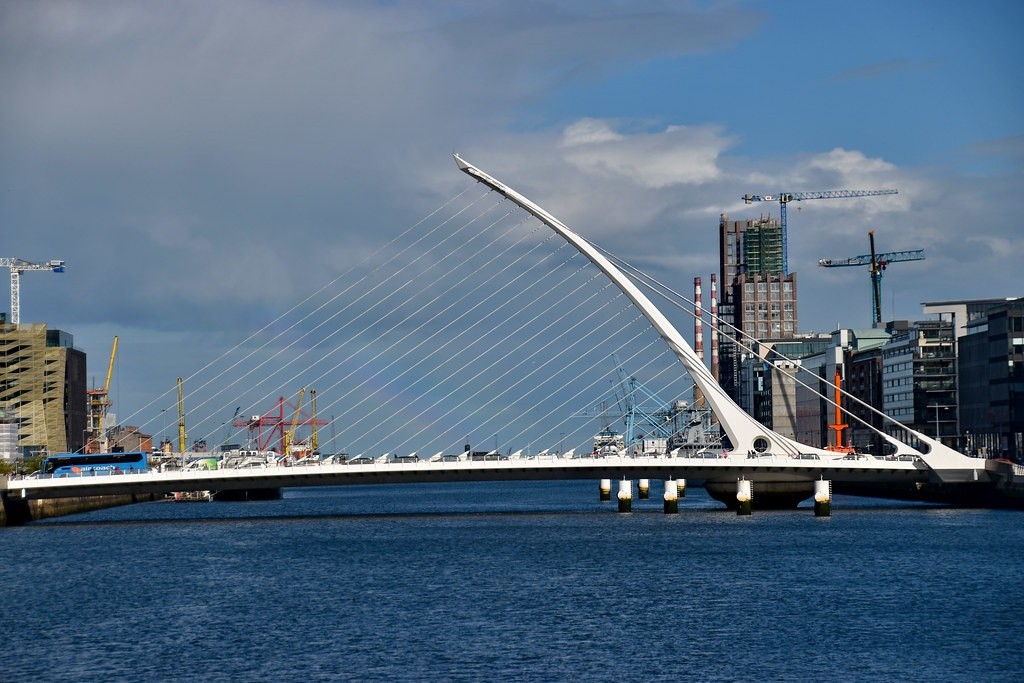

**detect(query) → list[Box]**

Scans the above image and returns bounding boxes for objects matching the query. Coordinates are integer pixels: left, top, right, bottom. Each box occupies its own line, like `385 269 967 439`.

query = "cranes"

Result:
819 229 926 328
567 354 672 459
0 256 67 329
222 386 337 463
105 335 119 391
741 188 900 277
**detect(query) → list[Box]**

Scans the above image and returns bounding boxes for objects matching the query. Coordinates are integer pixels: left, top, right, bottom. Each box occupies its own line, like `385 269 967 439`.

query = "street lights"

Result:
175 376 186 452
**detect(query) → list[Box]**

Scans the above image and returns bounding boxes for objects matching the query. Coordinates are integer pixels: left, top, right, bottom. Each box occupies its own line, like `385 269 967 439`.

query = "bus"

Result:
38 451 146 479
223 450 275 462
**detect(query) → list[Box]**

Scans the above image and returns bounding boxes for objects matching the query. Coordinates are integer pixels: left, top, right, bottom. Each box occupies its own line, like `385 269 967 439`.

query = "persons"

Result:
90 467 95 476
130 465 134 474
1 467 28 480
847 450 864 460
721 449 728 459
152 455 347 475
392 439 700 462
115 465 120 474
747 449 758 459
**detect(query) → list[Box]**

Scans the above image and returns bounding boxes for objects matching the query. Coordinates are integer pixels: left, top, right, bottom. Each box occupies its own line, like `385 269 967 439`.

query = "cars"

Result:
24 469 41 480
690 451 723 458
342 457 373 465
796 453 820 460
432 455 460 462
898 454 918 461
831 453 868 461
384 456 417 464
485 453 508 460
756 451 777 458
